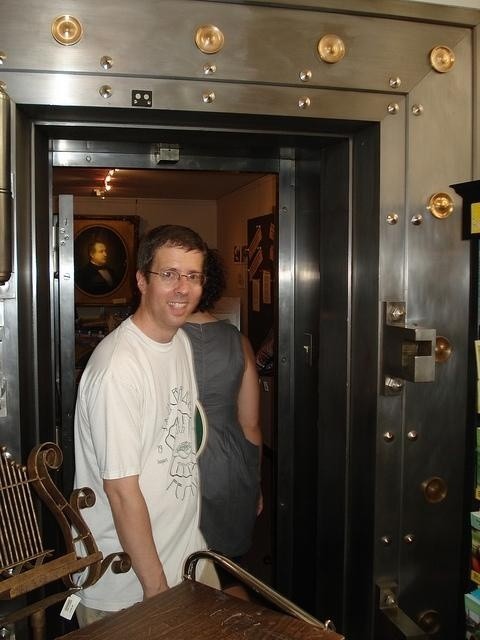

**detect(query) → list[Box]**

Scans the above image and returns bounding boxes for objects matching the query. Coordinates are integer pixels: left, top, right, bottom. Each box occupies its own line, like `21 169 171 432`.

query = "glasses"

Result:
144 267 207 289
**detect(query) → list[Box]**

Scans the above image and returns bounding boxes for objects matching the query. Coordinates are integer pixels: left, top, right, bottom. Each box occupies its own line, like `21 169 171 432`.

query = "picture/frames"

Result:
74 215 138 307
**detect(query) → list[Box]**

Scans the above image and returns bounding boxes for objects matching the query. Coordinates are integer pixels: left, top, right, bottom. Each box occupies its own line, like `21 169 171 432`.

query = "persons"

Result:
70 224 222 628
179 249 264 602
